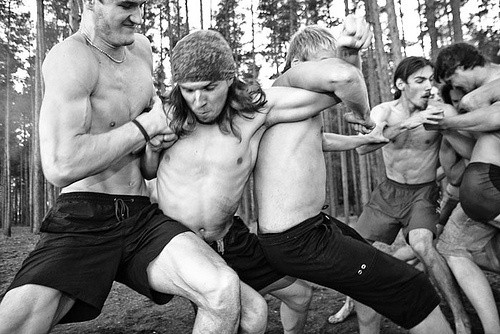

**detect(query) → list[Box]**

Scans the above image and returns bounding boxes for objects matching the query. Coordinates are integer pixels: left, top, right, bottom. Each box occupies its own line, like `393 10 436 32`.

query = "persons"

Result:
0 0 242 334
328 56 472 333
254 15 452 334
138 16 377 334
390 41 500 333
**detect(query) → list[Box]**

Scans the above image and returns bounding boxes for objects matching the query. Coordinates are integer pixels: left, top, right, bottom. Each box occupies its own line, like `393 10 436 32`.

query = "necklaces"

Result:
79 32 127 64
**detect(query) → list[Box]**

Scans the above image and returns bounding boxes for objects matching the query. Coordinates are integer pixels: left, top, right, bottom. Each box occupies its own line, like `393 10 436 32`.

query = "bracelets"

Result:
130 119 151 144
